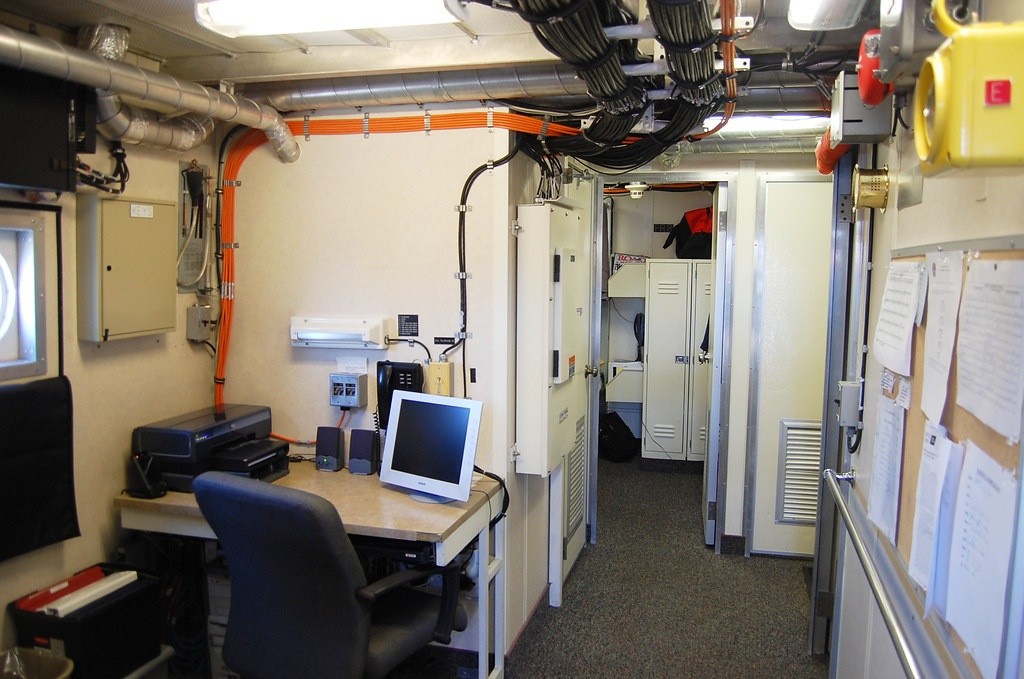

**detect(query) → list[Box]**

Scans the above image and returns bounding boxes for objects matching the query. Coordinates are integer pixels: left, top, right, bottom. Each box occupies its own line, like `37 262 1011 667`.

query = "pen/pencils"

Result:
132 456 155 495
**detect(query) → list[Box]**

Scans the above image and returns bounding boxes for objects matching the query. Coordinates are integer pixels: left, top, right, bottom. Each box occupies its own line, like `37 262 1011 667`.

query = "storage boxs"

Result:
7 563 176 679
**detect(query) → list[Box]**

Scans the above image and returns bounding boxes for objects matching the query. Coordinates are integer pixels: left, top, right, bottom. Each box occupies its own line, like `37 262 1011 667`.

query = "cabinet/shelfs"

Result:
605 258 713 461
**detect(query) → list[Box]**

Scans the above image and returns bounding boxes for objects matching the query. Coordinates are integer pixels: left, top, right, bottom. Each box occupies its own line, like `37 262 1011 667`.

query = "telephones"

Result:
633 312 645 347
374 360 426 429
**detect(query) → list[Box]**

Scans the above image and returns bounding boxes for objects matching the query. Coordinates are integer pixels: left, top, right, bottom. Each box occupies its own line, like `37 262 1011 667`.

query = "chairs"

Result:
194 471 469 679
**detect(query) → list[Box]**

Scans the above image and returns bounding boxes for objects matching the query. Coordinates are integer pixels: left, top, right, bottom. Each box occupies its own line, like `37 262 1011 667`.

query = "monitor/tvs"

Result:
380 390 483 504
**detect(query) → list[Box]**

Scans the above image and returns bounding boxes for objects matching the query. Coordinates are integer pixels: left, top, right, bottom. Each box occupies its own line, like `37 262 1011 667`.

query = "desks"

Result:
115 461 506 679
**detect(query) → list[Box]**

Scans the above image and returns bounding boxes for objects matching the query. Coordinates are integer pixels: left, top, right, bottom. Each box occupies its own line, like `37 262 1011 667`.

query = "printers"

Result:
133 403 290 494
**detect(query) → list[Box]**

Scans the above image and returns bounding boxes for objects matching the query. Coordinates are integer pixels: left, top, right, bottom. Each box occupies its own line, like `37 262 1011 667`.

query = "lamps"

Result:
193 0 470 38
702 111 831 135
291 317 389 351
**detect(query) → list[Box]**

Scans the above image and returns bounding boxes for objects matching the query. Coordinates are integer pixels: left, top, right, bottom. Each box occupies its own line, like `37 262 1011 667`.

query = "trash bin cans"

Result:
0 647 74 678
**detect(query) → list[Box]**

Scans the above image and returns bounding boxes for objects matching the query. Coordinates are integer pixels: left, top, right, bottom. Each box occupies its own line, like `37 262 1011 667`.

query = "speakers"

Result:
316 426 344 471
348 429 378 475
